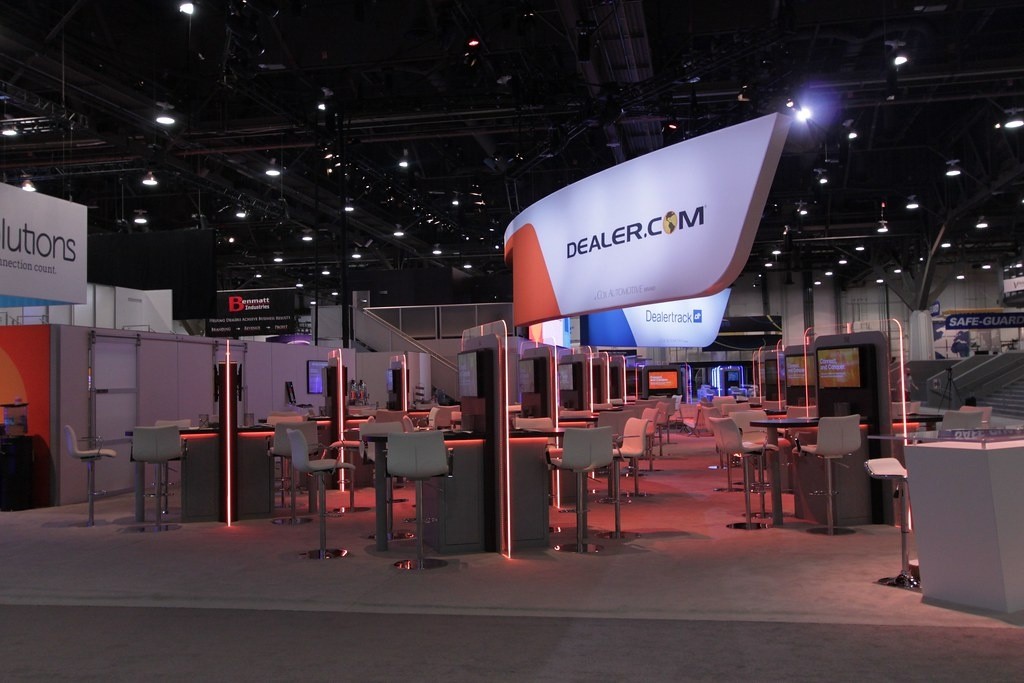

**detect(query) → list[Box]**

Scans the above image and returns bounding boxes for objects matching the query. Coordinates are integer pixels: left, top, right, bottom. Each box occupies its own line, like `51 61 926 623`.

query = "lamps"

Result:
21 179 36 191
393 223 404 236
274 252 284 262
399 149 409 168
142 168 158 186
906 194 919 209
945 159 961 175
266 158 282 176
351 247 361 258
432 243 441 255
1003 108 1024 128
877 213 889 233
134 209 147 224
738 84 750 101
976 216 988 228
155 101 176 125
669 111 679 129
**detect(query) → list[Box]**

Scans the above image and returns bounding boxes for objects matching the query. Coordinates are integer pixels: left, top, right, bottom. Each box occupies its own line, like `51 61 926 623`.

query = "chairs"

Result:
891 400 993 439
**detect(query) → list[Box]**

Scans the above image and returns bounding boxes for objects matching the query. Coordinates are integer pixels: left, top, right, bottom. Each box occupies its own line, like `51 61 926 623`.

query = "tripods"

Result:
937 371 963 413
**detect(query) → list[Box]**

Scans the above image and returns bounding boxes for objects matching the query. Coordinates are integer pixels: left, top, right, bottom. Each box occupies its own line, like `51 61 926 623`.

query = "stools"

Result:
264 402 463 571
511 384 818 556
864 457 919 593
61 424 116 527
797 414 861 535
122 420 191 532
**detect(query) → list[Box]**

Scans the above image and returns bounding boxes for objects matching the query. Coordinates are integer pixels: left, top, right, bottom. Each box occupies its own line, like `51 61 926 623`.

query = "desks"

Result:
750 403 762 408
750 418 821 530
510 429 567 438
763 408 787 415
364 425 487 553
893 414 943 429
124 426 276 435
593 407 623 412
866 429 1024 591
735 399 750 403
307 415 374 421
557 416 598 422
